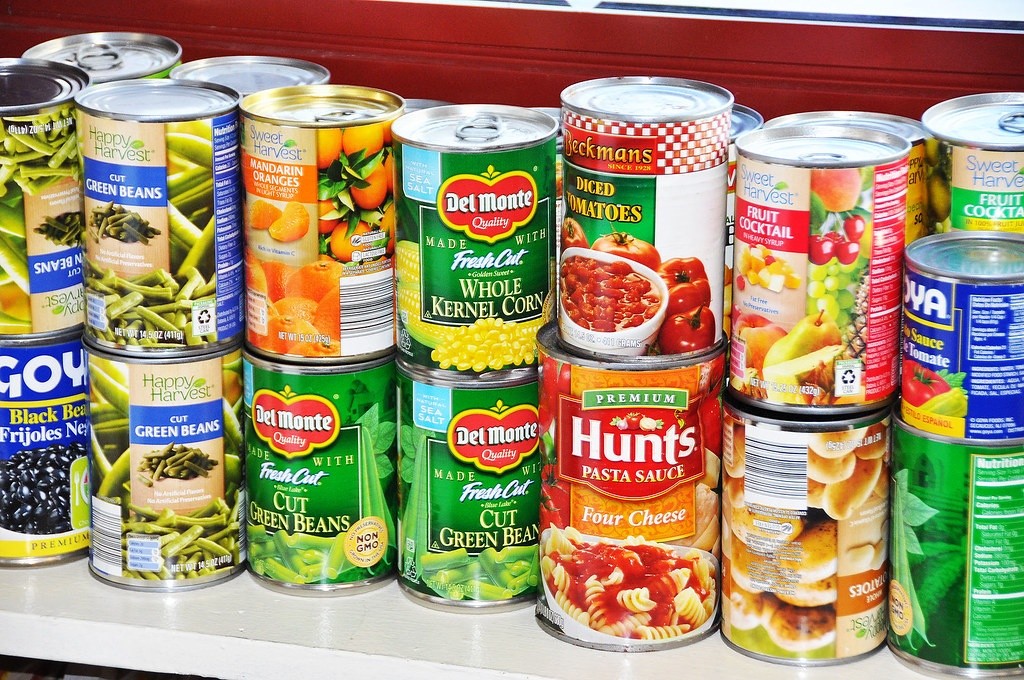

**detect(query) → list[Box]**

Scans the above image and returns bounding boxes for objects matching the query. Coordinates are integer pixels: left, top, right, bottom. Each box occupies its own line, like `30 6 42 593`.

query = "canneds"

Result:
0 28 1024 680
